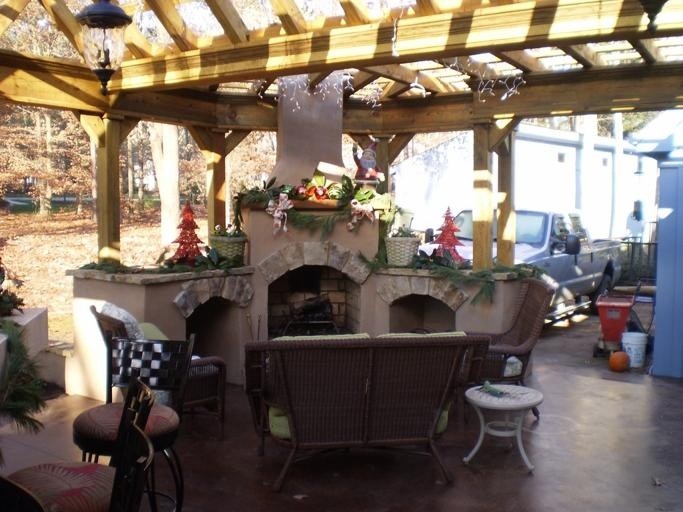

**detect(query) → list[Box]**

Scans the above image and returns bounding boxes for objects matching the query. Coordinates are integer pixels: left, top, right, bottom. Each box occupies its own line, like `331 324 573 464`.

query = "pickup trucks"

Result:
418 211 626 325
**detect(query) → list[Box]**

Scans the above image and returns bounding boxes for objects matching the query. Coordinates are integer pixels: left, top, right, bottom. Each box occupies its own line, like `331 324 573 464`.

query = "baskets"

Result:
210 236 246 259
385 236 420 267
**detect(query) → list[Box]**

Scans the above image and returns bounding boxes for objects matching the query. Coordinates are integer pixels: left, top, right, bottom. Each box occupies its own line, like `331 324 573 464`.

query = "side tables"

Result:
461 383 544 476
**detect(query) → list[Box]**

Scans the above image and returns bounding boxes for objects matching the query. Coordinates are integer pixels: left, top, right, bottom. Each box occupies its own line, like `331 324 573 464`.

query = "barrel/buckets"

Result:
621 333 649 368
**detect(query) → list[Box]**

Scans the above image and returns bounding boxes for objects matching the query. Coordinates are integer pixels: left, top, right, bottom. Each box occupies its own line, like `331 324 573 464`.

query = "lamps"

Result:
73 0 132 95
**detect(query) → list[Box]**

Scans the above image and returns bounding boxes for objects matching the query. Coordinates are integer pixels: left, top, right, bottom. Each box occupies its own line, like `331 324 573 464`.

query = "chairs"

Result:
90 305 226 442
466 277 555 421
0 473 45 512
613 240 657 332
72 329 197 511
8 406 155 512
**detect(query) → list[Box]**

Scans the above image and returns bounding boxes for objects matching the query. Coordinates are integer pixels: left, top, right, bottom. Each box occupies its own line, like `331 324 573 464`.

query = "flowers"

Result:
208 222 245 238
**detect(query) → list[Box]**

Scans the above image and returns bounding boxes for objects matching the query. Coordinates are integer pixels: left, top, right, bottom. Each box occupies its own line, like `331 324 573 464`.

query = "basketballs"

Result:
608 352 630 372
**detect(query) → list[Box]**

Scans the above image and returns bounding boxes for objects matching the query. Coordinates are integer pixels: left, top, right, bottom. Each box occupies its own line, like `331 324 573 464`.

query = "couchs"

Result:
243 331 491 491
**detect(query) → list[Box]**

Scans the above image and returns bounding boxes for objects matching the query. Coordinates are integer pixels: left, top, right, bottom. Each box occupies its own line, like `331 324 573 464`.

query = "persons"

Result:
352 143 385 189
626 201 644 236
